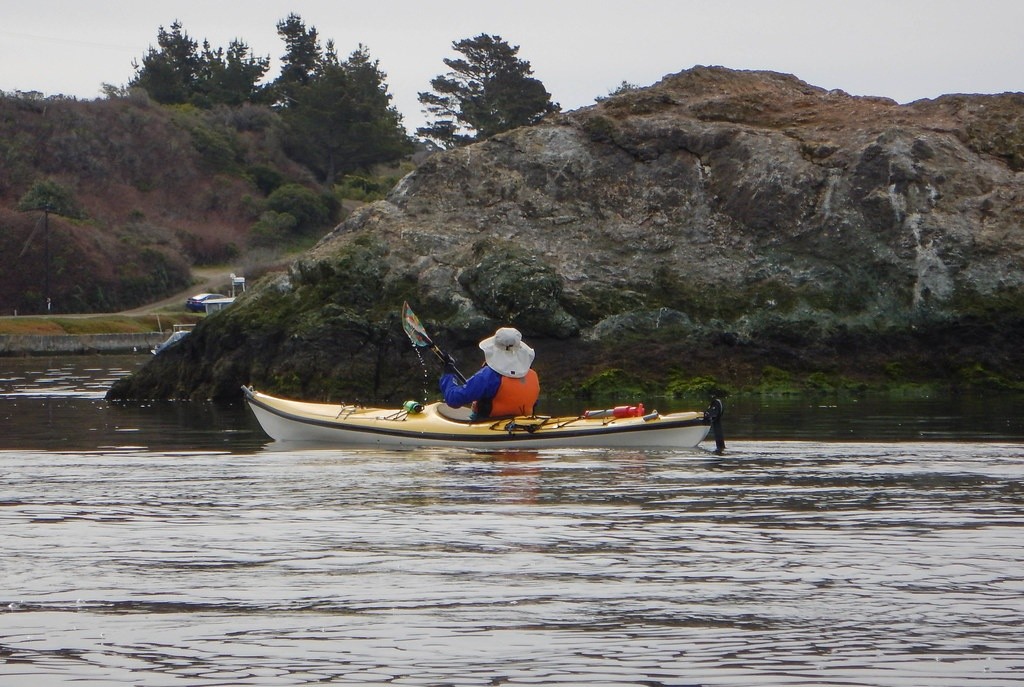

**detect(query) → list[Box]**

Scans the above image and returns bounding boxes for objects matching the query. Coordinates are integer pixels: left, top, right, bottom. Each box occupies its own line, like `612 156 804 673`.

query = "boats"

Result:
240 384 726 453
150 273 246 356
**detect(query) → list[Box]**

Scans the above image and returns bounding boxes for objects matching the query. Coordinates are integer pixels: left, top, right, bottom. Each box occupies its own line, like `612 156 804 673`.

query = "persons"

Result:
438 326 541 421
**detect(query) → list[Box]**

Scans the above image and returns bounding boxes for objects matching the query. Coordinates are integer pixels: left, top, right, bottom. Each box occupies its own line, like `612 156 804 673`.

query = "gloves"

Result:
441 354 455 370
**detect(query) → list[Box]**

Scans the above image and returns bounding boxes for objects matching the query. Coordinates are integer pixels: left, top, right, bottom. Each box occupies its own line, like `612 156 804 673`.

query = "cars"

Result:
186 293 229 311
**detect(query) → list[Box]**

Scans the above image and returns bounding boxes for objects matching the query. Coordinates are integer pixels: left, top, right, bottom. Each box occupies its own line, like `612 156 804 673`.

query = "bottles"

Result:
403 400 425 415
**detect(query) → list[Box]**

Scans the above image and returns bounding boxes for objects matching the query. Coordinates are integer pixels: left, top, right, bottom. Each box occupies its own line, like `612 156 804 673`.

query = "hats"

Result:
479 328 535 378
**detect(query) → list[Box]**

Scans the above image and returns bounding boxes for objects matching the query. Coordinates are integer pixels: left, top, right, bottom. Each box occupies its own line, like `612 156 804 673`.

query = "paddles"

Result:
402 298 467 383
